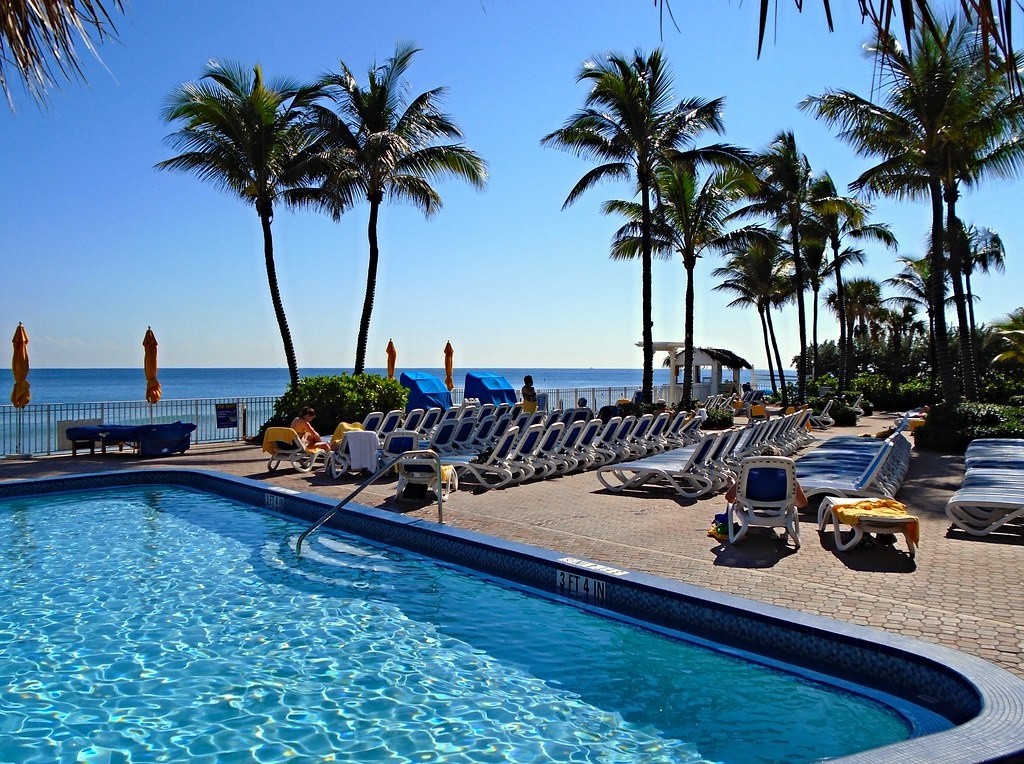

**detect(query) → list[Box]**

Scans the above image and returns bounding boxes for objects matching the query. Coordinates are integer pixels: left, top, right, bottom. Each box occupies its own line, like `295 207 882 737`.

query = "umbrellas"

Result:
443 339 454 391
385 338 396 380
10 321 31 455
143 325 161 425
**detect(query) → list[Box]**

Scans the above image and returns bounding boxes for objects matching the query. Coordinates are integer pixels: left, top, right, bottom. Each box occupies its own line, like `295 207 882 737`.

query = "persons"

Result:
680 380 756 427
724 445 808 511
654 398 676 427
578 397 588 408
897 394 970 418
290 405 332 452
329 422 368 453
521 375 537 415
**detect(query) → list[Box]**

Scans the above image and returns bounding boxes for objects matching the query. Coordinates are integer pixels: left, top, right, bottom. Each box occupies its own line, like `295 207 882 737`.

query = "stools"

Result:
263 389 1024 554
